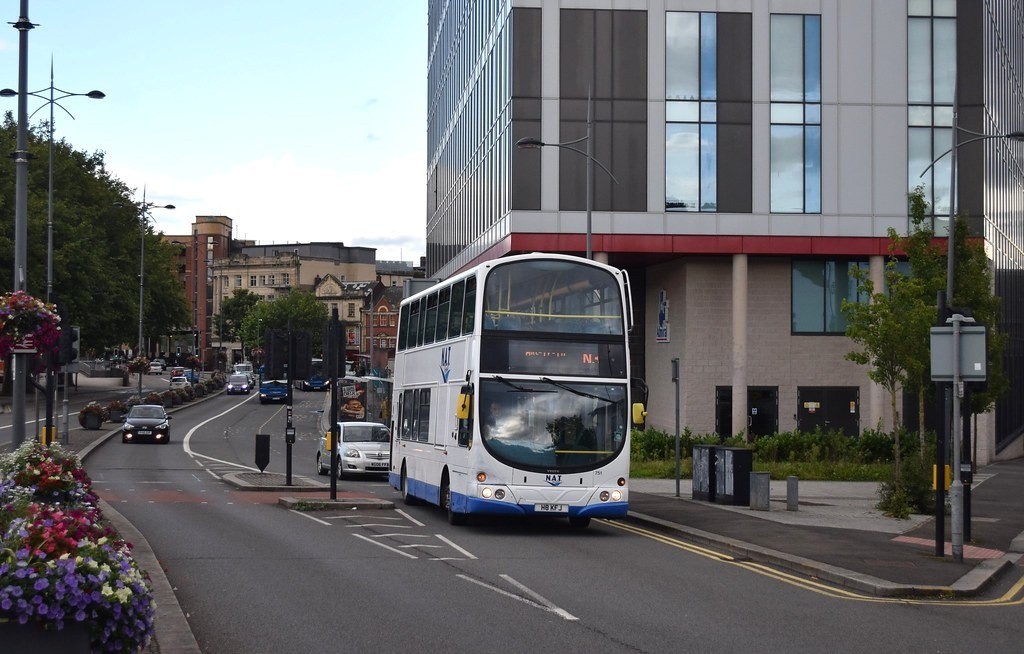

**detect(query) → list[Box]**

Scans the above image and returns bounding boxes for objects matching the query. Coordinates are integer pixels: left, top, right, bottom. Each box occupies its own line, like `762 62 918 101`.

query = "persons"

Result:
483 401 502 435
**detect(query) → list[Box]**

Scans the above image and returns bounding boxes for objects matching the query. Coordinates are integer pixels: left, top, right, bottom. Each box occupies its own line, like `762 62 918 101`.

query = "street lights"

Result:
202 259 240 373
172 228 219 390
256 319 263 369
917 109 1024 558
0 52 106 452
113 200 176 399
514 136 619 257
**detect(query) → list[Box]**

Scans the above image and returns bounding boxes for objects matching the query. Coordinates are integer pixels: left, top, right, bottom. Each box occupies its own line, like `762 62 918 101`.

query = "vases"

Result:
110 411 124 422
176 399 184 405
203 390 208 395
166 402 173 408
197 392 204 397
185 397 191 402
187 364 196 369
190 395 195 401
134 366 143 373
207 388 213 393
218 357 224 361
83 415 102 430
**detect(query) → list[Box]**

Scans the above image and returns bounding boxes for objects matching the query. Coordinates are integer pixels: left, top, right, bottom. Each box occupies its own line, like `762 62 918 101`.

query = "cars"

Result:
182 370 200 383
247 375 254 389
153 359 167 371
169 376 189 392
169 366 185 377
316 421 393 480
119 405 174 444
146 362 163 375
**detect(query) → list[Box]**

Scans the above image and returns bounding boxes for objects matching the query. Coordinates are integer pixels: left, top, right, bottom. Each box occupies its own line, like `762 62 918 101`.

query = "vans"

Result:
233 363 254 377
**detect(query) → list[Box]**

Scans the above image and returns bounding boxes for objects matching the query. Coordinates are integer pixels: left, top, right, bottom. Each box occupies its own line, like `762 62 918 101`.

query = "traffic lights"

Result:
924 388 937 436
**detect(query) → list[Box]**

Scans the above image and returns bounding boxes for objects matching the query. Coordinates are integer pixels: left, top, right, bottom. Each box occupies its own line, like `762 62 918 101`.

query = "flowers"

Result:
105 400 129 420
78 401 111 428
128 355 151 377
185 356 209 368
127 369 227 407
216 352 227 363
0 290 64 372
0 435 157 654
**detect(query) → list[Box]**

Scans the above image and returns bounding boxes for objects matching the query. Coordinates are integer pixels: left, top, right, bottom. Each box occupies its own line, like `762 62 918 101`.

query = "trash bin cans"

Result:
715 447 753 505
691 445 719 500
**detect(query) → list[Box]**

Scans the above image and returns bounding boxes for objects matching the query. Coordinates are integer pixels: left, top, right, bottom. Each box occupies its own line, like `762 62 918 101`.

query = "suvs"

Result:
227 374 250 395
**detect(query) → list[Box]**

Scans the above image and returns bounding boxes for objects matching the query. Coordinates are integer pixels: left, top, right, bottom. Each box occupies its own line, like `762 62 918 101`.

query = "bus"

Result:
258 364 295 403
292 358 331 392
388 252 650 530
